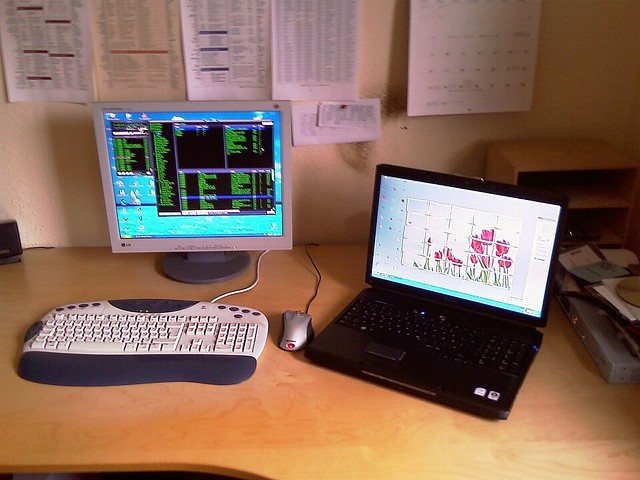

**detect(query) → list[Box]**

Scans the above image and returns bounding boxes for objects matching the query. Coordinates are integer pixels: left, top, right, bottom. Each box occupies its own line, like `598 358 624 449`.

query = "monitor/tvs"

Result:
91 100 295 285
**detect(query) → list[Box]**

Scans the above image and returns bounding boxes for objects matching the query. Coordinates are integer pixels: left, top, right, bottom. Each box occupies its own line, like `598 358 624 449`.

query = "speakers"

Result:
0 216 24 265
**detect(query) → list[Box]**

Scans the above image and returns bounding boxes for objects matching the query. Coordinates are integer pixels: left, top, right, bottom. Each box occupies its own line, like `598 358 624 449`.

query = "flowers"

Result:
422 228 514 290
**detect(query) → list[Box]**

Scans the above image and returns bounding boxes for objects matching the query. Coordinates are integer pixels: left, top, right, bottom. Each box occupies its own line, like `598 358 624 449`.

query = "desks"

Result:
0 137 640 480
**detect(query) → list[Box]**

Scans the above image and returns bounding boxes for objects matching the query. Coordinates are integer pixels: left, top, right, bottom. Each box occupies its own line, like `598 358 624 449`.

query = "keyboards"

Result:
16 298 269 387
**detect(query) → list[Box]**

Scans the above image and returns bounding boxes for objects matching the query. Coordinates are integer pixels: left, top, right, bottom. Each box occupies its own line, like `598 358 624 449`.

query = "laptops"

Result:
302 162 570 420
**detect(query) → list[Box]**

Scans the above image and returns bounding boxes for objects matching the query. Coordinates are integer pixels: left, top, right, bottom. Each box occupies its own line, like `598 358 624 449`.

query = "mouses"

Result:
275 306 315 351
